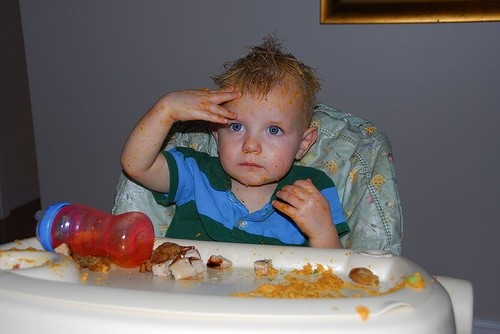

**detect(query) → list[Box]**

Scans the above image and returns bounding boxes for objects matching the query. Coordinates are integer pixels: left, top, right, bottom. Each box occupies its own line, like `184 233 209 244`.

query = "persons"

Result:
119 50 349 251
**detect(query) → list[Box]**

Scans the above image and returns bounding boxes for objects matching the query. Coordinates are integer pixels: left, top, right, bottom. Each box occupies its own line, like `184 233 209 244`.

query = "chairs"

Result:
0 103 473 334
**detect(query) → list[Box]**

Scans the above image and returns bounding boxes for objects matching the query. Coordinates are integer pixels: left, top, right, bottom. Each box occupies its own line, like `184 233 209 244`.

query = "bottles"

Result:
35 201 155 268
